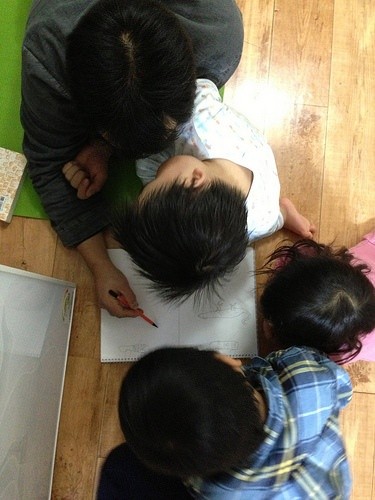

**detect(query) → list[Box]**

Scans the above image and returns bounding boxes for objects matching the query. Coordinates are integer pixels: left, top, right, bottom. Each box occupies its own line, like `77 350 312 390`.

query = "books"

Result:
100 248 260 362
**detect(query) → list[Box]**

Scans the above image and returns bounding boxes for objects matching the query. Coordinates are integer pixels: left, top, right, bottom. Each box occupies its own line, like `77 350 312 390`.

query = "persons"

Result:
101 345 352 500
20 0 243 319
251 229 375 372
111 78 316 311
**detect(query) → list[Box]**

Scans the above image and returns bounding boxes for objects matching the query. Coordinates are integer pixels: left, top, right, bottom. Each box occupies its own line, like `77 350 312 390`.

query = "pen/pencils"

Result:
108 289 159 328
